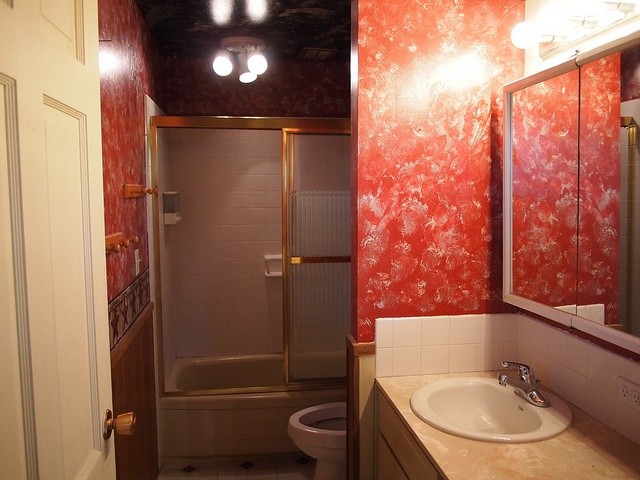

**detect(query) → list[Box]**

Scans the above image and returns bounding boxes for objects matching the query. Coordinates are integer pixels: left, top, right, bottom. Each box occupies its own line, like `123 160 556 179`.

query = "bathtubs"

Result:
162 352 347 457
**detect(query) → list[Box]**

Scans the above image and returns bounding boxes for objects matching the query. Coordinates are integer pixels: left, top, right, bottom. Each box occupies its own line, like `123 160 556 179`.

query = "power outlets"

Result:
615 376 639 412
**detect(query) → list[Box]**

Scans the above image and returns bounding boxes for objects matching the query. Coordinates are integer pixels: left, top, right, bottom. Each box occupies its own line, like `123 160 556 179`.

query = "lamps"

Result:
212 36 269 83
511 7 625 49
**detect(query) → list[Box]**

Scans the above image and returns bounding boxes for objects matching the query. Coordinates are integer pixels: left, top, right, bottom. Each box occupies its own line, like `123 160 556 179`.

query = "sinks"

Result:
408 377 573 442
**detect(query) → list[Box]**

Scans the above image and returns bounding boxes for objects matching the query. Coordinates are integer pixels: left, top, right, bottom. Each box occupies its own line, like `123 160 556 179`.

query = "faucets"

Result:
502 358 535 383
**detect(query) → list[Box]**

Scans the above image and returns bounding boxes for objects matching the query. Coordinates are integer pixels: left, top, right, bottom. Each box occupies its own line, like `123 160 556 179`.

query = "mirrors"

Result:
503 29 639 354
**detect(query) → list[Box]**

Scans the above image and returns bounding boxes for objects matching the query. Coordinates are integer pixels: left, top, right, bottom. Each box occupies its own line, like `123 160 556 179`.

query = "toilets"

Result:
287 402 347 479
